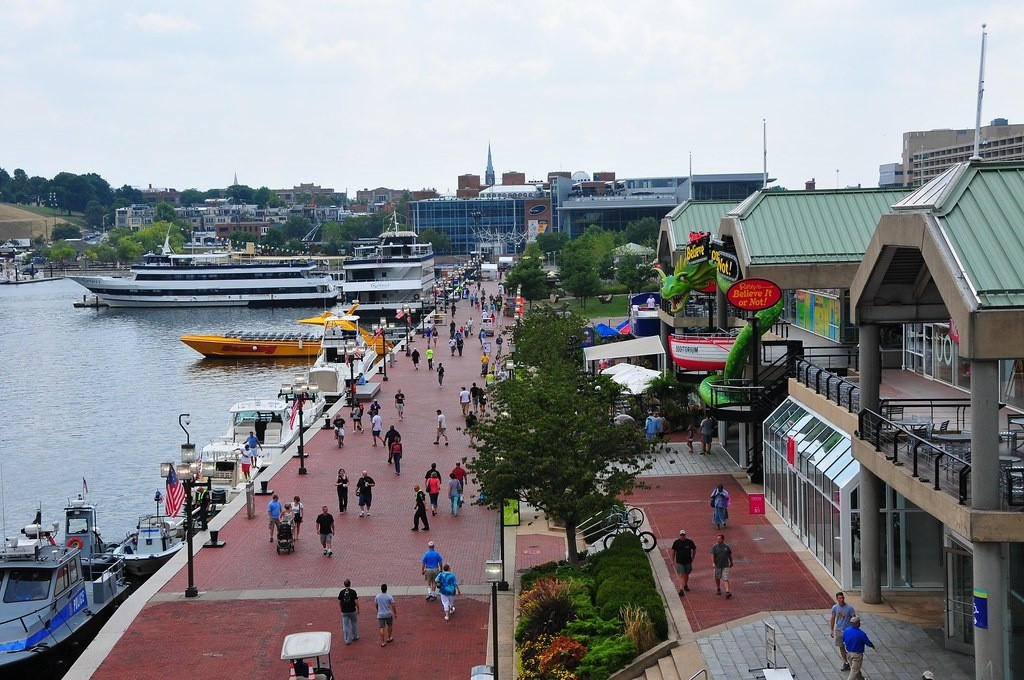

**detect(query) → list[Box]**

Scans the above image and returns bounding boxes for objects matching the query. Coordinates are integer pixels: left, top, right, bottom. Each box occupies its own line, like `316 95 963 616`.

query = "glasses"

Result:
680 533 685 537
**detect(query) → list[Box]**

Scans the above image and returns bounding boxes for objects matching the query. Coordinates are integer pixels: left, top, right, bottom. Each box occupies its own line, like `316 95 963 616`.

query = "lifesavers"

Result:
414 294 420 300
67 537 82 549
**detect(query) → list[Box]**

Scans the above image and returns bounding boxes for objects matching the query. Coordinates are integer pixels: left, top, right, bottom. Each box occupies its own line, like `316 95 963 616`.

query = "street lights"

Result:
433 283 438 314
278 373 318 474
160 443 216 598
396 304 417 357
484 559 503 680
419 293 428 338
541 249 562 267
506 359 514 381
493 452 509 591
372 317 395 381
337 339 365 417
440 278 449 313
451 254 485 306
102 213 111 233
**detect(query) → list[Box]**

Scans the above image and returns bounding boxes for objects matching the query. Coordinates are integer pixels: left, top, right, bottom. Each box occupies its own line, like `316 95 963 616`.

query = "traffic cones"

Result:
289 663 297 680
307 661 316 680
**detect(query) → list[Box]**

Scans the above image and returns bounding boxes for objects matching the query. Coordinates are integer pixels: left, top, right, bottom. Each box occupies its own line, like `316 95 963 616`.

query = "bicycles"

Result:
599 502 644 532
602 519 657 552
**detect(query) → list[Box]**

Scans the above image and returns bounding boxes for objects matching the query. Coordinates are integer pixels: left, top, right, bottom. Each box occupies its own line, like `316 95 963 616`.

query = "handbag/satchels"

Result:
426 485 430 492
711 489 717 508
437 582 442 588
297 516 302 523
457 480 462 494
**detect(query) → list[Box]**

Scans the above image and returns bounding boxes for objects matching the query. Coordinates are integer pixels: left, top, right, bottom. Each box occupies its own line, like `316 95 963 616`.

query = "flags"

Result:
289 392 306 430
83 479 88 493
165 465 185 519
396 309 405 319
345 340 360 366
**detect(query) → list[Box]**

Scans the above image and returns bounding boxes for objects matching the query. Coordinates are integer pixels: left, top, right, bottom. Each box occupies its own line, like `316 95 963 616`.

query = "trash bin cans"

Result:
503 497 520 527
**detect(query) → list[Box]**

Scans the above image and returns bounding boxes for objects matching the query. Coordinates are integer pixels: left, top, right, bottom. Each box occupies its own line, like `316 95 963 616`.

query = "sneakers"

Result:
323 548 327 554
360 511 365 517
367 510 370 516
328 549 333 554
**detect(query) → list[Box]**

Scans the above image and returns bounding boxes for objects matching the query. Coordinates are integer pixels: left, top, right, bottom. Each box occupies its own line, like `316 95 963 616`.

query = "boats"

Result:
64 223 339 308
220 399 306 468
0 475 133 676
189 436 262 511
315 310 378 382
331 209 439 322
179 300 395 359
112 488 190 576
306 365 346 406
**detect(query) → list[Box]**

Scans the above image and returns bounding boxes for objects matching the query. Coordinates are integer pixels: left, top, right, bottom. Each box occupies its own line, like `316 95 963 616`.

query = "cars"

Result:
480 318 495 337
83 231 101 241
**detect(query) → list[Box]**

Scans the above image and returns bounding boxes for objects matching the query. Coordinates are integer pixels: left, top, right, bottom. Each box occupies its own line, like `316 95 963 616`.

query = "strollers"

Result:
277 520 295 555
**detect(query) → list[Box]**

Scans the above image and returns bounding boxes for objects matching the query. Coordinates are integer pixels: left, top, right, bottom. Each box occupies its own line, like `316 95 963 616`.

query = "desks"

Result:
964 457 1021 502
1010 418 1024 451
895 418 930 454
930 433 971 485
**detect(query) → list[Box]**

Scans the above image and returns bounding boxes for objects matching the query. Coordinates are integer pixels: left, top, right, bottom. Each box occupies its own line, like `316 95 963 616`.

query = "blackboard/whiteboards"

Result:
764 621 776 667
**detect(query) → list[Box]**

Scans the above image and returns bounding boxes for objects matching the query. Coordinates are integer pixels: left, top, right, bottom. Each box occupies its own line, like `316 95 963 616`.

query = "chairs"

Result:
876 405 1024 509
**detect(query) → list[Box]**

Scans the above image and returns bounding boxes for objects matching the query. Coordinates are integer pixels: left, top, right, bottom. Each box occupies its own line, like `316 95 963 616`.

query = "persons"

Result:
195 486 211 531
458 281 507 322
645 410 666 450
687 413 713 454
411 317 507 388
332 388 406 476
337 579 360 644
598 359 608 369
411 462 467 530
671 530 696 596
709 534 734 599
646 294 655 307
267 469 376 556
422 541 461 620
236 431 262 479
710 483 730 530
831 592 875 680
374 583 397 647
356 373 366 385
433 383 488 448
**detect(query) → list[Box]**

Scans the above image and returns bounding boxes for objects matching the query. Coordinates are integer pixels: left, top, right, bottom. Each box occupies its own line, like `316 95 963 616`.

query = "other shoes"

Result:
444 616 449 621
433 441 439 444
726 592 732 599
450 607 455 614
386 638 393 642
411 526 418 531
716 527 719 529
432 510 435 516
445 441 449 446
339 507 347 514
684 585 690 591
434 512 437 515
381 642 387 647
678 590 685 597
841 662 850 671
689 447 711 455
716 589 721 595
723 524 728 529
426 593 437 599
422 526 429 530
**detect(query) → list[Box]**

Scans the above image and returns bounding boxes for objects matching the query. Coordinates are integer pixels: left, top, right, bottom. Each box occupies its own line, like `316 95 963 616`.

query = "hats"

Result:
679 530 686 535
850 616 860 624
648 411 663 417
923 671 934 679
